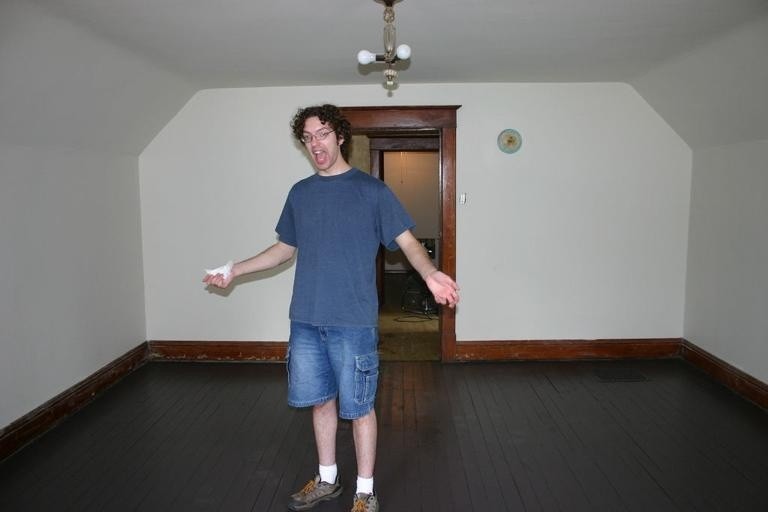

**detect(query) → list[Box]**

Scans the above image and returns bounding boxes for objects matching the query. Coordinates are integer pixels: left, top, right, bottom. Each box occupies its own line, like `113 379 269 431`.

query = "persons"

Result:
199 103 460 511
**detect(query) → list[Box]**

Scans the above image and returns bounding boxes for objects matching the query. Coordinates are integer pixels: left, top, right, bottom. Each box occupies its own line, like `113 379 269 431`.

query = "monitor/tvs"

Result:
416 237 439 264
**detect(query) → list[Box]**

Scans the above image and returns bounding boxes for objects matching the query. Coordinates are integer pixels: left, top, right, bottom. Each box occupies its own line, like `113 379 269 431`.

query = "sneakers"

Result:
287 474 343 511
350 488 379 512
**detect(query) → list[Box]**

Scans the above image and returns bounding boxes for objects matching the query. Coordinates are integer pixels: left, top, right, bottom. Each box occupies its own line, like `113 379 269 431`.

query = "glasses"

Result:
300 129 334 143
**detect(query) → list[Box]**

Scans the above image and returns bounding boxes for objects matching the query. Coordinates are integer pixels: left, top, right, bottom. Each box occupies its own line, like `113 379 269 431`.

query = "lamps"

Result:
354 0 413 88
